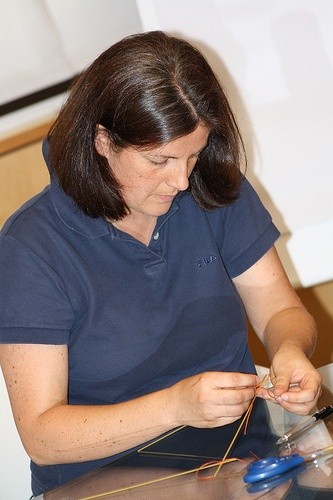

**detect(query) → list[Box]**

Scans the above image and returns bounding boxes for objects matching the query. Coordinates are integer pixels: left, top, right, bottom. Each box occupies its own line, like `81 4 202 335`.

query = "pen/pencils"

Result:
275 404 333 445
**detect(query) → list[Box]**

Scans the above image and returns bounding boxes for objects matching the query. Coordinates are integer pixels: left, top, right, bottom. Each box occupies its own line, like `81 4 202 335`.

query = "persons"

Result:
0 26 322 496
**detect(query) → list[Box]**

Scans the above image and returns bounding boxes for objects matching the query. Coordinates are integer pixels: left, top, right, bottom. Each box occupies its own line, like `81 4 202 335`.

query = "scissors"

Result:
244 446 333 480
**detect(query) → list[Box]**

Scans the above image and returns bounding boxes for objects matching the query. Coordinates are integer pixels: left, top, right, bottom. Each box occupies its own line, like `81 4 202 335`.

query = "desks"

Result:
34 361 333 500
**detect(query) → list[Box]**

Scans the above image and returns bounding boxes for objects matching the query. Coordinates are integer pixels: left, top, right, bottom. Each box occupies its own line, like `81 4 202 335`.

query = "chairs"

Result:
248 278 333 374
